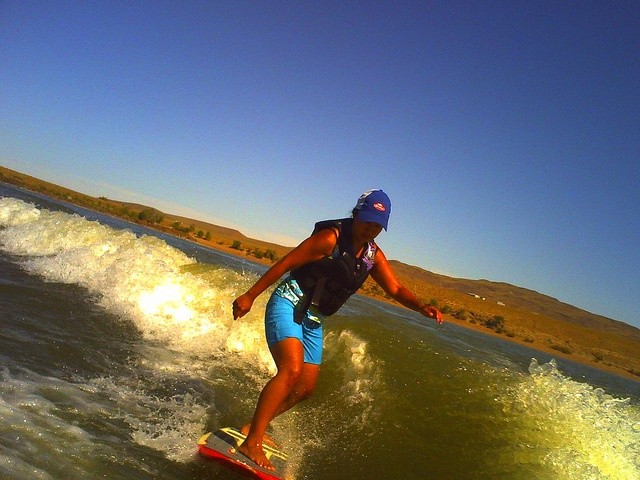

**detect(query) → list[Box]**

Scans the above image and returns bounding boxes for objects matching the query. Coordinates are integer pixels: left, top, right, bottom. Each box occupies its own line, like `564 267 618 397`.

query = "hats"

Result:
354 189 391 232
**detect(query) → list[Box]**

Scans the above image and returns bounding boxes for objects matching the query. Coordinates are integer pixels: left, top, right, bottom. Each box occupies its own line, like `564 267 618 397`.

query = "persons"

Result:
233 189 443 472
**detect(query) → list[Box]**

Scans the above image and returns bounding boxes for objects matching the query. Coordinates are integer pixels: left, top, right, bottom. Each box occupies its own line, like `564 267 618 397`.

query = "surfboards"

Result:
197 423 286 480
